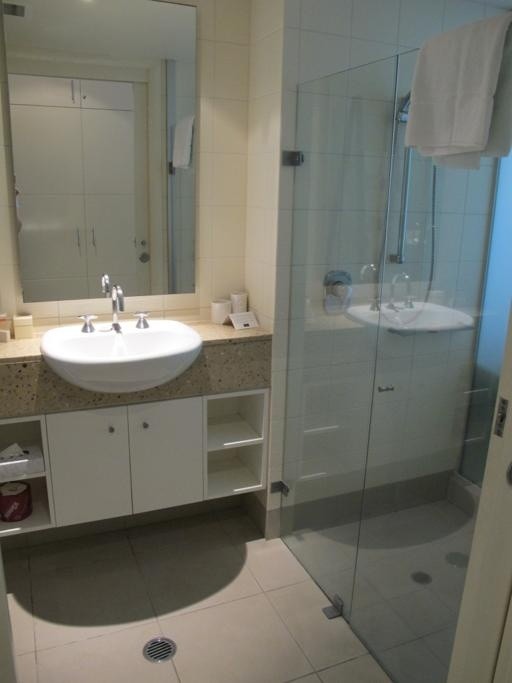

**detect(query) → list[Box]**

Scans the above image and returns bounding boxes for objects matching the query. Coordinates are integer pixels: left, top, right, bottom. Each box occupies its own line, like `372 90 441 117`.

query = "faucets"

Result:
359 264 381 311
387 272 414 310
111 283 124 334
99 273 110 298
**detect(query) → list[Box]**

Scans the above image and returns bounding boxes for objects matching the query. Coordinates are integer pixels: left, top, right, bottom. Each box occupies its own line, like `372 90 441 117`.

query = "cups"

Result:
210 299 231 325
231 292 248 314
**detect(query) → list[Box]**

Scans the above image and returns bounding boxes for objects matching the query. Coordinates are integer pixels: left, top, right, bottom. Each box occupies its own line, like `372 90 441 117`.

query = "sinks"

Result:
345 302 476 357
39 319 202 393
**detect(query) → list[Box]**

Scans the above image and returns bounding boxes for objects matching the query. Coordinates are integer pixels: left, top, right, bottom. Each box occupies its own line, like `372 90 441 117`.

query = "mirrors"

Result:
1 0 198 308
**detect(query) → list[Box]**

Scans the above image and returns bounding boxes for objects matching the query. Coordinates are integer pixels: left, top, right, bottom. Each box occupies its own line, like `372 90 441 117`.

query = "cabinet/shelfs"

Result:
8 74 150 300
46 398 205 526
0 421 51 534
204 393 265 498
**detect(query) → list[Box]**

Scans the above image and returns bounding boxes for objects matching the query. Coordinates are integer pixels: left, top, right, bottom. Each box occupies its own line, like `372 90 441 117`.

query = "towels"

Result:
171 114 195 170
403 12 512 170
